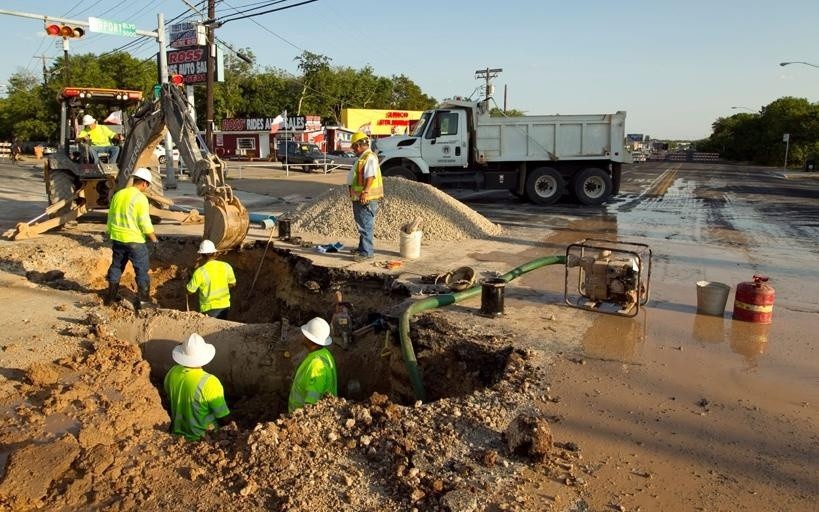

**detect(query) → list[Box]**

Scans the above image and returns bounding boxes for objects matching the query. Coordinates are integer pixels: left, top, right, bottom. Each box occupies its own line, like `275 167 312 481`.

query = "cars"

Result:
280 140 333 174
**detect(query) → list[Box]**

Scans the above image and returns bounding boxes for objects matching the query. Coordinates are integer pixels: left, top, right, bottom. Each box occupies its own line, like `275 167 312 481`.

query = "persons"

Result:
186 239 237 320
104 167 162 308
288 316 338 413
74 114 124 164
347 131 385 261
164 333 234 442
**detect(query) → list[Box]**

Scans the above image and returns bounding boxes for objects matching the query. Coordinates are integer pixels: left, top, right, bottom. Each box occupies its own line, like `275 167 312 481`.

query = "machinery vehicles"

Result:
1 86 251 257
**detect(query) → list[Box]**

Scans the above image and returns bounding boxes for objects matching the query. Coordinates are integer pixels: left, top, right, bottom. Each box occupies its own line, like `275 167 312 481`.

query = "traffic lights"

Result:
172 74 185 85
45 21 85 40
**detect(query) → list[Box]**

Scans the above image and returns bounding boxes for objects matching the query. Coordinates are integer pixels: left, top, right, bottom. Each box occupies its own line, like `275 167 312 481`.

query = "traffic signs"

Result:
89 17 137 38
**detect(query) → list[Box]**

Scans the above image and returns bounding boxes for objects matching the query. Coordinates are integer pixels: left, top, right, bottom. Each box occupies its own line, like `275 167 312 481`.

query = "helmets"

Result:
197 239 218 254
301 317 333 347
350 131 368 145
172 333 216 368
82 114 96 125
129 167 153 184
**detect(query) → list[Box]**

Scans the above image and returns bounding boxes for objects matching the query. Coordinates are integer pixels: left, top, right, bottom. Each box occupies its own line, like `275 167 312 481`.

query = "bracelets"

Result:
362 191 369 194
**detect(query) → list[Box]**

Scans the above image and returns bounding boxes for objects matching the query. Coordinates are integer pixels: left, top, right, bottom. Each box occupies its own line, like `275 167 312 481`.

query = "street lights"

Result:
779 59 819 74
729 104 759 113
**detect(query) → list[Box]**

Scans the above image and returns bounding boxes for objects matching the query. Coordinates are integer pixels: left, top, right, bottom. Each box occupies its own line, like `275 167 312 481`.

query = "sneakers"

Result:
356 254 374 261
350 248 361 253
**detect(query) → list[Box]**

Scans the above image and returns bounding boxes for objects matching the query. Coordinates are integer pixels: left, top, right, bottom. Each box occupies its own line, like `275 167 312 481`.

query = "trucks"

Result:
374 102 627 206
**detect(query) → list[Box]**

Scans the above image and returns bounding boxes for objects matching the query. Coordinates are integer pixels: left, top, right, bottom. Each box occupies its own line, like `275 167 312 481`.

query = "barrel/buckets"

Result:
400 230 423 259
697 281 730 316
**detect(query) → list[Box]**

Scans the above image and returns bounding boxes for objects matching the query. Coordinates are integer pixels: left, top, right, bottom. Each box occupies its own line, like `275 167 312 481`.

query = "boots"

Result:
136 282 155 308
105 281 122 302
